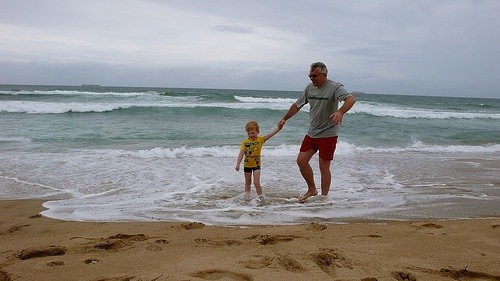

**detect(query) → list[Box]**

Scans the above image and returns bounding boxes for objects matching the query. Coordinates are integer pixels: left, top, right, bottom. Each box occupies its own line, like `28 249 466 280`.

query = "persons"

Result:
276 61 357 203
235 119 283 206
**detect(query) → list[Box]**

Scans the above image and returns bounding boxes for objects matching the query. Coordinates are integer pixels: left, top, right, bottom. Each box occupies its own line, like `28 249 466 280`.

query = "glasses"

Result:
308 73 324 78
